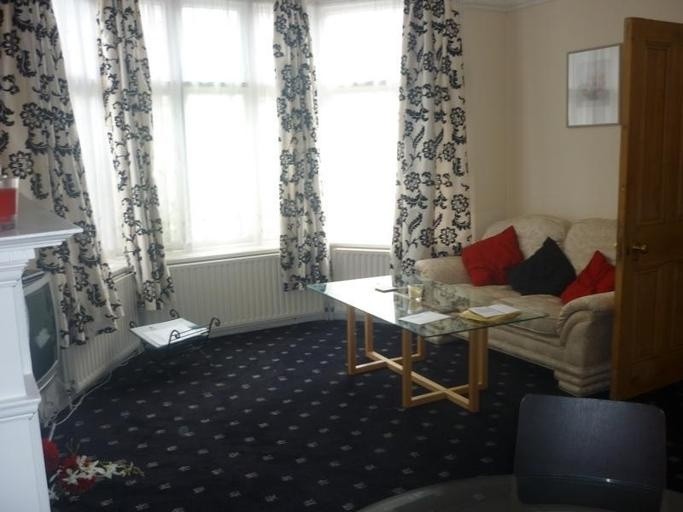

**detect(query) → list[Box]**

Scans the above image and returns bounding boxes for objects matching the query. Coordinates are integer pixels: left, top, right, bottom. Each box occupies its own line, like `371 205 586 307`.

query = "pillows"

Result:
459 222 525 287
556 246 616 309
498 233 578 298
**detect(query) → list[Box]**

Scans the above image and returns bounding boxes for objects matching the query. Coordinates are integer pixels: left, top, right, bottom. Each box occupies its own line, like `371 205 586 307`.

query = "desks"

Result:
125 305 221 381
352 467 683 512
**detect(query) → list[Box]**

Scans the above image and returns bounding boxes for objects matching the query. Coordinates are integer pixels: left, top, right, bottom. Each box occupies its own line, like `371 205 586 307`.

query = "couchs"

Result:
414 213 628 398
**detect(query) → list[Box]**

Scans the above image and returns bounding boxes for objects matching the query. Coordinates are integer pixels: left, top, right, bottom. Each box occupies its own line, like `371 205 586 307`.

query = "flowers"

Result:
43 451 146 503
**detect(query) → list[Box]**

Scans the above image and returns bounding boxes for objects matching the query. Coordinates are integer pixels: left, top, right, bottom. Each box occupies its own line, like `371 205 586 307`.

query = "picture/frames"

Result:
565 41 623 129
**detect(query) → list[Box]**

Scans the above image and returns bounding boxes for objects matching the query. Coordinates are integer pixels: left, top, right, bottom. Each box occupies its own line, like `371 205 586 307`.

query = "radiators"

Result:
51 267 150 398
138 251 328 339
325 244 396 323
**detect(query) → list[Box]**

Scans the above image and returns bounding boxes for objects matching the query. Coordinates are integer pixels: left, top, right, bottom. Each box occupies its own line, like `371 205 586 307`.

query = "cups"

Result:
406 283 424 301
406 302 424 314
0 175 18 229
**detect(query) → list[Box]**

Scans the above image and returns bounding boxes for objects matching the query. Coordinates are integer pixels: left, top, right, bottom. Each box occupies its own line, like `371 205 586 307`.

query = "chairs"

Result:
510 385 670 490
